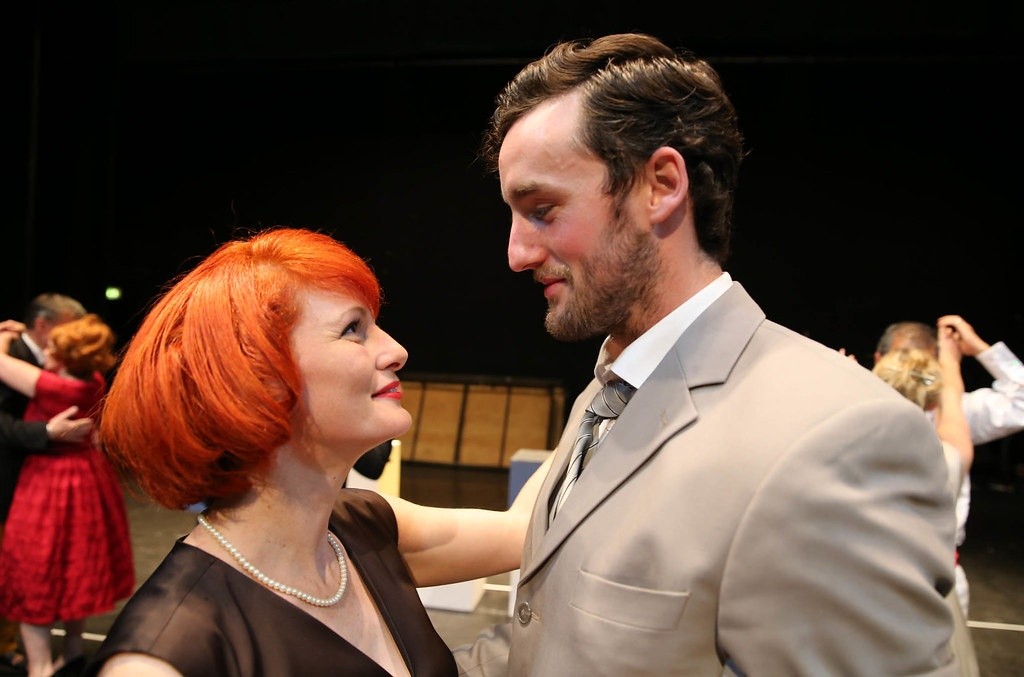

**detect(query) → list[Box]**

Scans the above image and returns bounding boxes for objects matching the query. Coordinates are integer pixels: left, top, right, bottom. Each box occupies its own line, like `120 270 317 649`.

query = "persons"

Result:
91 230 558 677
837 316 1024 677
453 33 958 677
0 296 136 677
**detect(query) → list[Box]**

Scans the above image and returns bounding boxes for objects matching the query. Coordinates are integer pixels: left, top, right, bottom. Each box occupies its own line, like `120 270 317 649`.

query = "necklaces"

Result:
198 515 347 606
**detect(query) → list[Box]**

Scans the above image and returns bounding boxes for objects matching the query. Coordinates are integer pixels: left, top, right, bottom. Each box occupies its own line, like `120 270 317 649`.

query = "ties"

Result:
548 377 633 531
